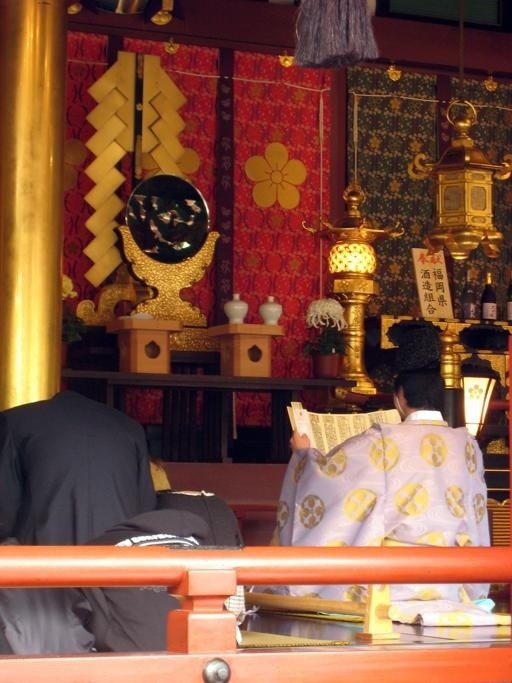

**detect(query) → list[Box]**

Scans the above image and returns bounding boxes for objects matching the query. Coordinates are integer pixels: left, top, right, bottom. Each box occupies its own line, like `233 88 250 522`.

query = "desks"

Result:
62 365 357 464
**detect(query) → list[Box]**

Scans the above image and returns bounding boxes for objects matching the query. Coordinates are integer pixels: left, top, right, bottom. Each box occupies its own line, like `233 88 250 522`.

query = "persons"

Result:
250 368 511 628
155 488 248 644
0 537 98 655
80 509 211 653
1 391 159 545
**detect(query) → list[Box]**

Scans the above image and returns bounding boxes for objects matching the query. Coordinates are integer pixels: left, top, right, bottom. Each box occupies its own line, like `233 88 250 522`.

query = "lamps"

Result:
300 174 405 401
405 0 511 262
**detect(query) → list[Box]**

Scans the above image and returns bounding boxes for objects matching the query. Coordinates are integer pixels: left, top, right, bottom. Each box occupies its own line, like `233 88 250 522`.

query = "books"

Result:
285 398 404 456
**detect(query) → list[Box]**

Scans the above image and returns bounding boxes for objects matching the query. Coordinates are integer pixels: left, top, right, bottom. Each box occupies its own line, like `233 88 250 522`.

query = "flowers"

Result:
62 270 89 344
301 295 349 357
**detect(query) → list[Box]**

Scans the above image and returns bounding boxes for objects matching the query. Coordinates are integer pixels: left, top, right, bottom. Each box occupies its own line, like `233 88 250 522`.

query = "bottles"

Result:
462 269 478 319
506 278 512 324
261 294 282 325
482 272 498 319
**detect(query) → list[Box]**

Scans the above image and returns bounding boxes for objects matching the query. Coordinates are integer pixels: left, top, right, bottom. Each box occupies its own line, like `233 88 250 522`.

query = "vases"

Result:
257 294 284 325
62 340 71 365
310 352 343 379
